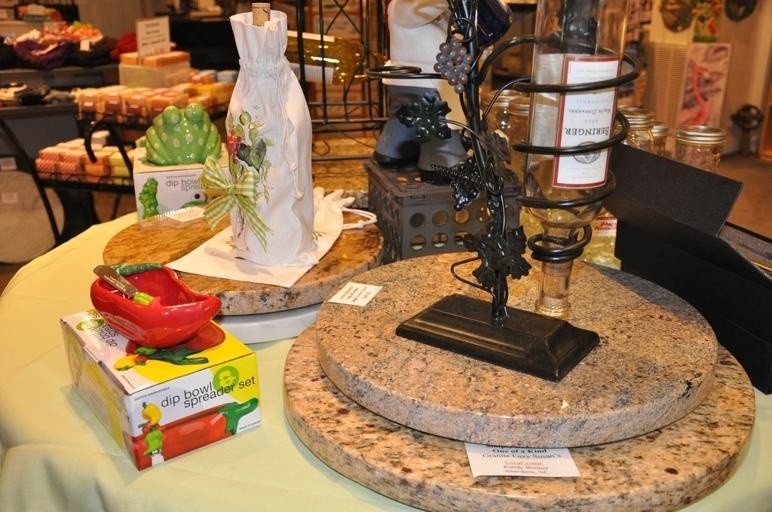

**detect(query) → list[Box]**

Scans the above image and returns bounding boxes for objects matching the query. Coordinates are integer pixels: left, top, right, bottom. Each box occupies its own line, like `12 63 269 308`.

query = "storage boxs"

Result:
132 142 230 222
58 307 263 471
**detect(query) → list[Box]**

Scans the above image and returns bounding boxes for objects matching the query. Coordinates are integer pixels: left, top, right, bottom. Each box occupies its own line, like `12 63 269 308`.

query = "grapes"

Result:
435 39 473 94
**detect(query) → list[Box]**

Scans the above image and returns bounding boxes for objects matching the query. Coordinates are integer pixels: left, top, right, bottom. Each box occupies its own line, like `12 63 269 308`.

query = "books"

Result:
190 11 222 17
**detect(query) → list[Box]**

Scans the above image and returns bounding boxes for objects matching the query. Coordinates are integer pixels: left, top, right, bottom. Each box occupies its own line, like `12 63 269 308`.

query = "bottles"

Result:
522 0 628 319
283 30 388 86
482 88 726 265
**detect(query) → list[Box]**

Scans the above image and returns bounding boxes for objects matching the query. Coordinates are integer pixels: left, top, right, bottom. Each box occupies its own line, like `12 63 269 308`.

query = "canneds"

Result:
482 89 728 173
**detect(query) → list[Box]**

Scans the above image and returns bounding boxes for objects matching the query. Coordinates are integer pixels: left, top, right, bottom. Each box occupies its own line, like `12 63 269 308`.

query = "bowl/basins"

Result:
89 263 222 348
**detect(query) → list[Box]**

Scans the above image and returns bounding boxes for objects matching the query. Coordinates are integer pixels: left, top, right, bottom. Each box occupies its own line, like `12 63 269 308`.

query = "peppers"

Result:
123 398 259 470
112 320 226 370
